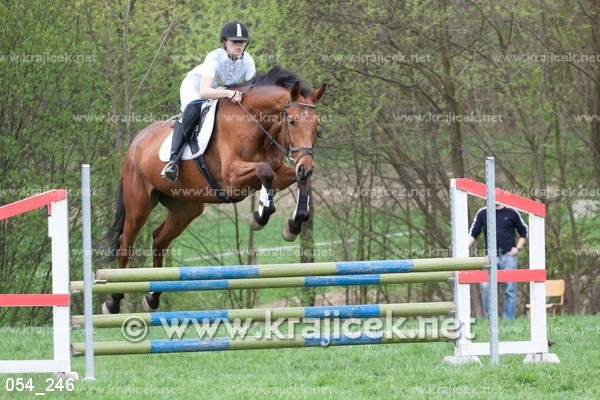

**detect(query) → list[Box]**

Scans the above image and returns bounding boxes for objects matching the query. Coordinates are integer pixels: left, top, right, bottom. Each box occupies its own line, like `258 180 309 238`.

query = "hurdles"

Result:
0 157 560 381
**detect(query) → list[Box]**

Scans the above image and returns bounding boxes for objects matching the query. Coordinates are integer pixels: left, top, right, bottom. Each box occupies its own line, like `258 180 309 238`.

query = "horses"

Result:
97 64 327 314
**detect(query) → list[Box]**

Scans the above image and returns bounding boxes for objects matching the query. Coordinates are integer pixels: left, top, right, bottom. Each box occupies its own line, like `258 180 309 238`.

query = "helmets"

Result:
220 21 248 41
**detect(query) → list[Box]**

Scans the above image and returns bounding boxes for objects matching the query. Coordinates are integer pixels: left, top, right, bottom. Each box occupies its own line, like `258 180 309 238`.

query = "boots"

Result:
162 120 185 183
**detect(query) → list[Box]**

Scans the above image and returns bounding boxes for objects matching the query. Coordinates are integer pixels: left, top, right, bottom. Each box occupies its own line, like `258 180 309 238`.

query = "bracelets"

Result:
229 91 237 100
516 247 520 252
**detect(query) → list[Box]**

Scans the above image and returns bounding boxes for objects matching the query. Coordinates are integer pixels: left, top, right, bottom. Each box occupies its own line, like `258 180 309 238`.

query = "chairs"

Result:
525 279 565 317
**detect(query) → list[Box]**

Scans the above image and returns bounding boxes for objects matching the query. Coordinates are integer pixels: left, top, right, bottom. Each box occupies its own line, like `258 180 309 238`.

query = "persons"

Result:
469 186 528 322
163 20 257 184
